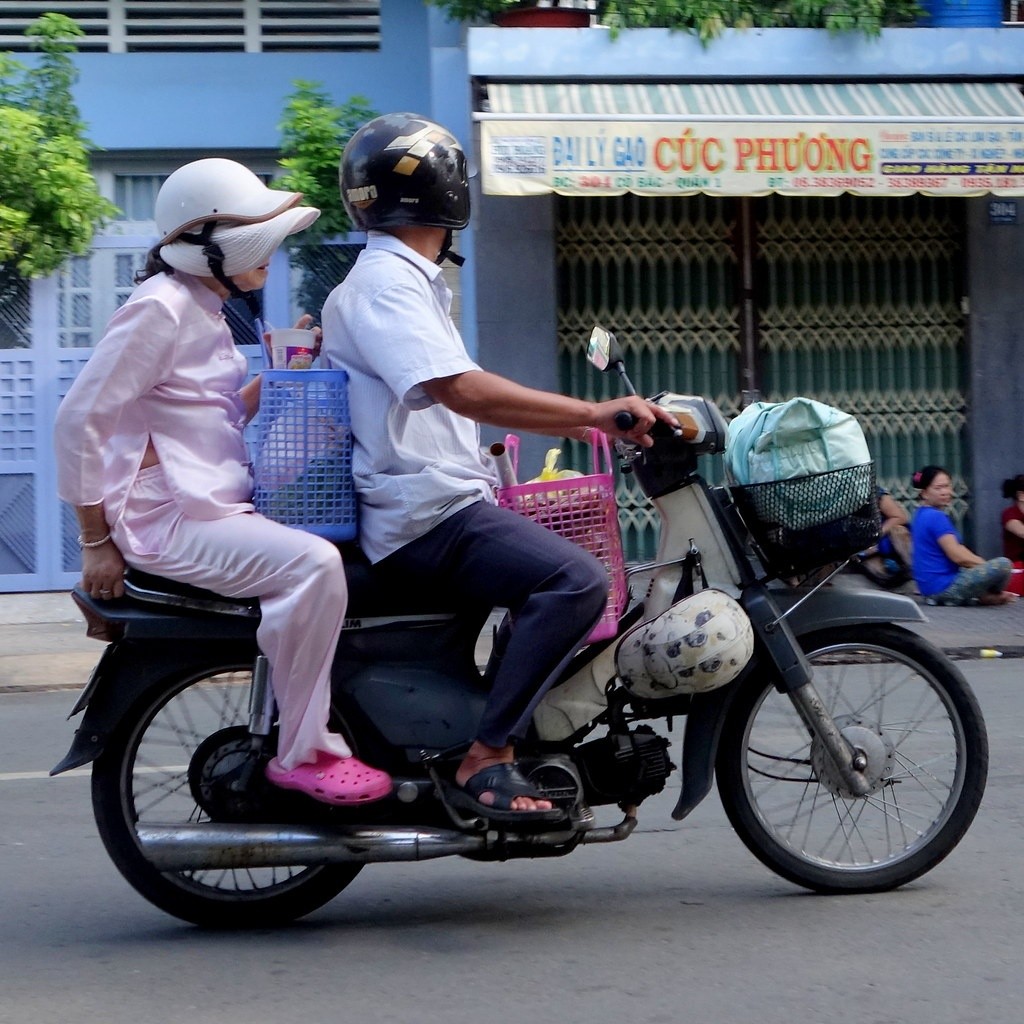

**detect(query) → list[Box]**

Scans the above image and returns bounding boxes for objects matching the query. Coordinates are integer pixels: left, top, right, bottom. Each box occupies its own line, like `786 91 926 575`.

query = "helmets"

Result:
336 111 479 229
154 157 304 245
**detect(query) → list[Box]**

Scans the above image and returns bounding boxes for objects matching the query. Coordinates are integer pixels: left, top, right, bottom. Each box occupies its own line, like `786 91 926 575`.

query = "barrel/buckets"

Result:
1003 563 1024 596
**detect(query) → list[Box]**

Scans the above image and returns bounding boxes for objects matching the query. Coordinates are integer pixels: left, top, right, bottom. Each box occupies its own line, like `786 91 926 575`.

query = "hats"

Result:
158 205 322 277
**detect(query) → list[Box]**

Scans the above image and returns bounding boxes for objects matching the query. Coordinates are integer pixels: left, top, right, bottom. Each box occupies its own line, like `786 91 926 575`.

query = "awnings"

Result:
479 81 1024 207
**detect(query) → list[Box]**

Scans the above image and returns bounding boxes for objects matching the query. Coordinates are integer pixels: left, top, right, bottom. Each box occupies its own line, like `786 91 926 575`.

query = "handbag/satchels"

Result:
723 396 873 530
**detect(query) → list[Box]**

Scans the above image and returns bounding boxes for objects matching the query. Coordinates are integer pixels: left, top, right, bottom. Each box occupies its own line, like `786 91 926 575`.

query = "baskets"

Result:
495 428 630 644
728 461 884 581
240 367 357 539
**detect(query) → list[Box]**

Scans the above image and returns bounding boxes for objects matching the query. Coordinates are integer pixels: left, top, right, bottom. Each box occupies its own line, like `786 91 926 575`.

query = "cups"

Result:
271 329 316 386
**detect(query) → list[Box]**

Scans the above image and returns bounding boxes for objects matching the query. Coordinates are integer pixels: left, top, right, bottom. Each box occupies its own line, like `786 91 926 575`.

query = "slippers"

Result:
445 759 564 821
265 756 394 805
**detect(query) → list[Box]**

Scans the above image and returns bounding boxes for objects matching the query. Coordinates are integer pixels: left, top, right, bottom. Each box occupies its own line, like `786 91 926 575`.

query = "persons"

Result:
865 466 1024 604
317 112 688 823
54 157 394 806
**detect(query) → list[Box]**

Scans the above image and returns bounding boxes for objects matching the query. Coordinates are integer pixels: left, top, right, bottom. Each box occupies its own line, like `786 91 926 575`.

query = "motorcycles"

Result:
46 325 989 934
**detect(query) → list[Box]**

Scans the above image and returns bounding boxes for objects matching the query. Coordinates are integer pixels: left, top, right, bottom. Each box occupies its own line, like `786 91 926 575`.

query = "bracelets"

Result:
77 534 109 548
